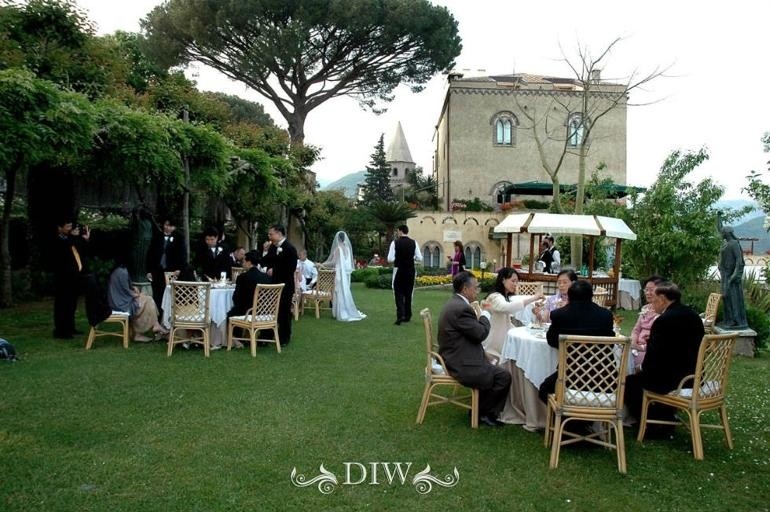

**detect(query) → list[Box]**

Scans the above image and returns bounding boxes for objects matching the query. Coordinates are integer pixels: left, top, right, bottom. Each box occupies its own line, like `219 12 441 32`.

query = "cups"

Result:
220 271 227 283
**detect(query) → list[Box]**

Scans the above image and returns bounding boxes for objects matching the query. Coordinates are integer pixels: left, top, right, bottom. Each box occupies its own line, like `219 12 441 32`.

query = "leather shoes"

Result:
480 416 504 426
134 327 169 343
396 316 410 325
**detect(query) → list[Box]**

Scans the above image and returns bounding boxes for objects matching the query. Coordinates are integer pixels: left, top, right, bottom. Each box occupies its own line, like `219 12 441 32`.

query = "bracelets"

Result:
539 295 543 299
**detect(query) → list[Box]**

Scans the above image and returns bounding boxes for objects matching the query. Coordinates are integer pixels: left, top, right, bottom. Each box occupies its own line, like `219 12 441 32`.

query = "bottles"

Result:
579 264 589 277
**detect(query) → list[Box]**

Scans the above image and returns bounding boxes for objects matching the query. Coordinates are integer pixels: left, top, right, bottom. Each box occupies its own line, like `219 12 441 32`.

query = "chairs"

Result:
83 262 336 358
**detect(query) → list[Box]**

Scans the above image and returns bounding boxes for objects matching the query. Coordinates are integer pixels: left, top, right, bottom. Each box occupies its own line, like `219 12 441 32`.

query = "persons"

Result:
386 225 424 325
479 266 544 370
716 225 749 331
446 240 466 279
621 281 708 445
368 253 382 267
537 241 555 272
320 230 368 323
622 274 668 431
536 280 615 454
39 214 321 355
533 269 577 326
545 234 561 274
435 268 513 430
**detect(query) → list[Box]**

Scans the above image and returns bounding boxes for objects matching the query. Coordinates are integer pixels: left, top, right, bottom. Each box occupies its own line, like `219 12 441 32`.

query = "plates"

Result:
523 323 547 333
